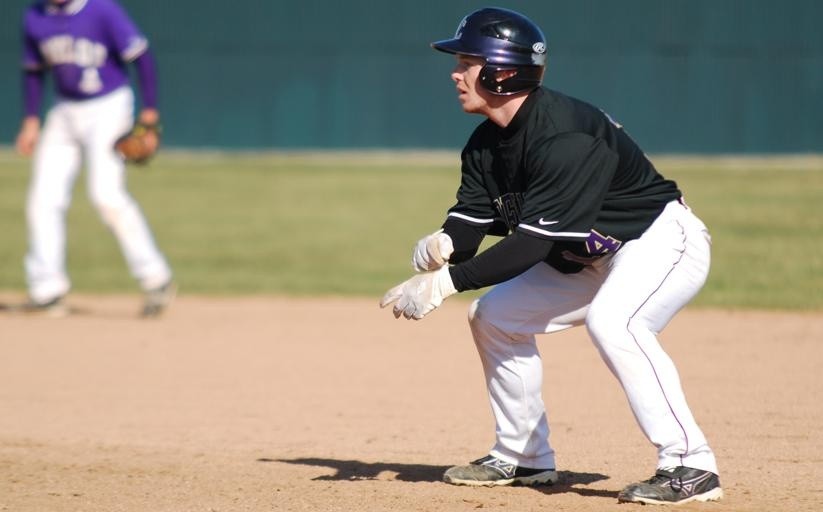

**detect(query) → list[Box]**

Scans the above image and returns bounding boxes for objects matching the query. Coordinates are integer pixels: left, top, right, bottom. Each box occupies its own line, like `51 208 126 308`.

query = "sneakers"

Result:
133 281 176 321
616 464 724 506
21 297 70 321
444 453 561 488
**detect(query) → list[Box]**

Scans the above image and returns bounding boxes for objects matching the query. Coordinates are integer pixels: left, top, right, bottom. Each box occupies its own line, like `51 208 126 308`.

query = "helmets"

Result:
430 5 547 94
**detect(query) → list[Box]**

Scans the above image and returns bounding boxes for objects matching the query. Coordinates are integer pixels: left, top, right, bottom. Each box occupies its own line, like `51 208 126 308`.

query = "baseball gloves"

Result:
114 123 158 164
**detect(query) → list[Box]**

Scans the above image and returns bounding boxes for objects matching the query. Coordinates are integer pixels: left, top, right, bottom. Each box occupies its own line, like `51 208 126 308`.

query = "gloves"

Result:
378 269 455 322
412 231 451 271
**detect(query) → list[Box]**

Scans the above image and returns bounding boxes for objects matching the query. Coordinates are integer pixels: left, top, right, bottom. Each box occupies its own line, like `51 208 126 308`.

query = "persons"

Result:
380 6 727 505
15 0 183 320
